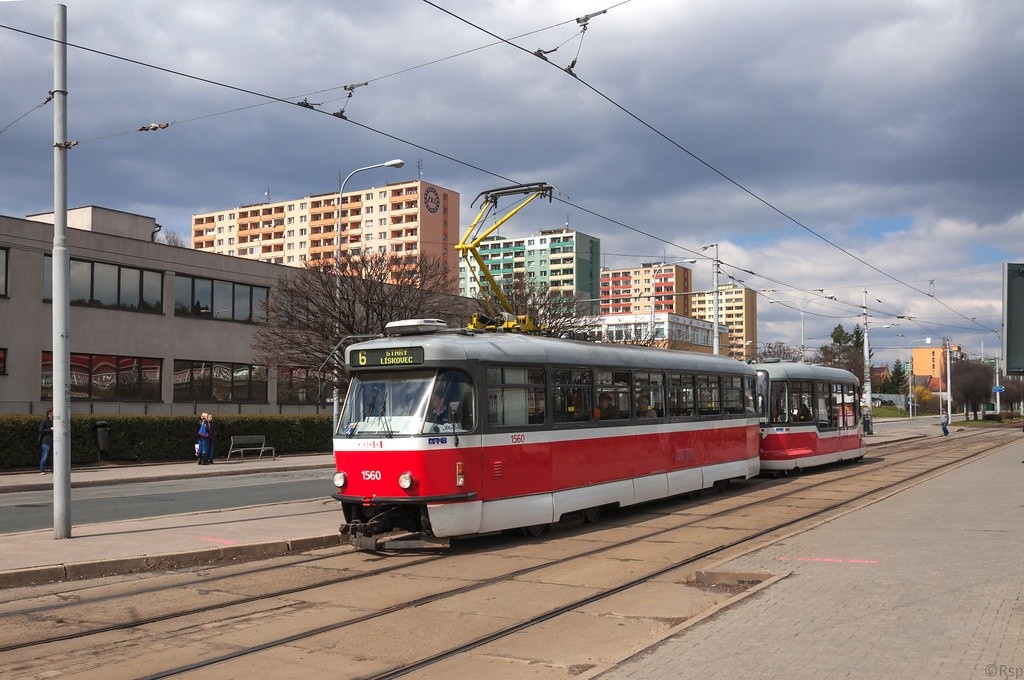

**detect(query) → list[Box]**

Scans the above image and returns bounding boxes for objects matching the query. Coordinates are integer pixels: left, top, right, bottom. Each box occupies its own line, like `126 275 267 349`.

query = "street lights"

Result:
860 325 891 408
331 156 402 440
747 340 766 359
906 336 932 419
770 294 835 365
652 258 696 345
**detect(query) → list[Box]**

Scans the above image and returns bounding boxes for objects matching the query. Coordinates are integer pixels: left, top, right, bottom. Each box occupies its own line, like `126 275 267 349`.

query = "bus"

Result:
317 317 868 553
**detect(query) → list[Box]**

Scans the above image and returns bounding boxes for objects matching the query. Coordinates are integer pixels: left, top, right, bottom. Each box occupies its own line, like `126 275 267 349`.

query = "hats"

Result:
597 393 612 402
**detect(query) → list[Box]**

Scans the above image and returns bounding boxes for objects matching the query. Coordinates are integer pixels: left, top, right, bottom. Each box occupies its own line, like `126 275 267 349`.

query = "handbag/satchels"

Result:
36 431 44 447
195 444 199 456
198 424 210 437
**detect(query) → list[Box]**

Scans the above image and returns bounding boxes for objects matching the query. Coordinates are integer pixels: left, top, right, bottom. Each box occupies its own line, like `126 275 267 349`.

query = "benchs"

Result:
227 435 275 463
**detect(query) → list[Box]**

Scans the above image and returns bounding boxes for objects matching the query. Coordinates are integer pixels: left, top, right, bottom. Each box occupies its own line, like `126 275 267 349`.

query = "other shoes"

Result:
948 433 951 436
40 471 45 475
197 458 214 465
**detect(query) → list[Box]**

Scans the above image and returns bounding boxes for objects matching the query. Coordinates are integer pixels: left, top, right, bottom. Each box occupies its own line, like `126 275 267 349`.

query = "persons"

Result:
428 394 456 425
596 394 619 419
208 415 215 464
941 408 950 437
638 395 657 418
798 404 811 421
39 408 54 474
198 413 209 464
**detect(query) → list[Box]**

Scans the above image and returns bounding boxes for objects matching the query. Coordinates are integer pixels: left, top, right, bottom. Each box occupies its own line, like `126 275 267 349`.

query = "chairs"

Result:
527 408 740 425
